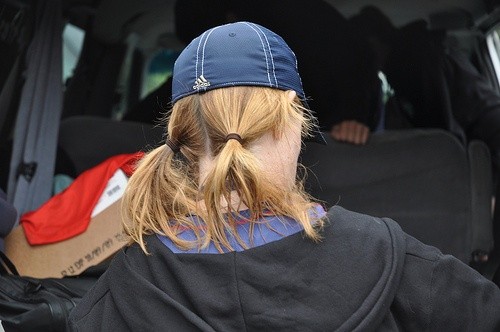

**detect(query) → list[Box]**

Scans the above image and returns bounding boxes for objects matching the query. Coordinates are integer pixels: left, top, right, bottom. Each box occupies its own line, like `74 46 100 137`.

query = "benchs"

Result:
57 116 474 265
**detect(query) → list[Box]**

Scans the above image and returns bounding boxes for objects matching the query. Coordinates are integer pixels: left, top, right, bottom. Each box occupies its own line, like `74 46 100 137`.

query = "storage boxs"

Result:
0 167 135 278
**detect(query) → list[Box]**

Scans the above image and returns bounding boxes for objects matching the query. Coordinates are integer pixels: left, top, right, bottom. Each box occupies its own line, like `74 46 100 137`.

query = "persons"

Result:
68 21 500 332
121 1 383 141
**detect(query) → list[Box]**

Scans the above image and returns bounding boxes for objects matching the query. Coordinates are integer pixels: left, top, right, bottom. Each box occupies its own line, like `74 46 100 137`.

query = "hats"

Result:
171 21 327 146
